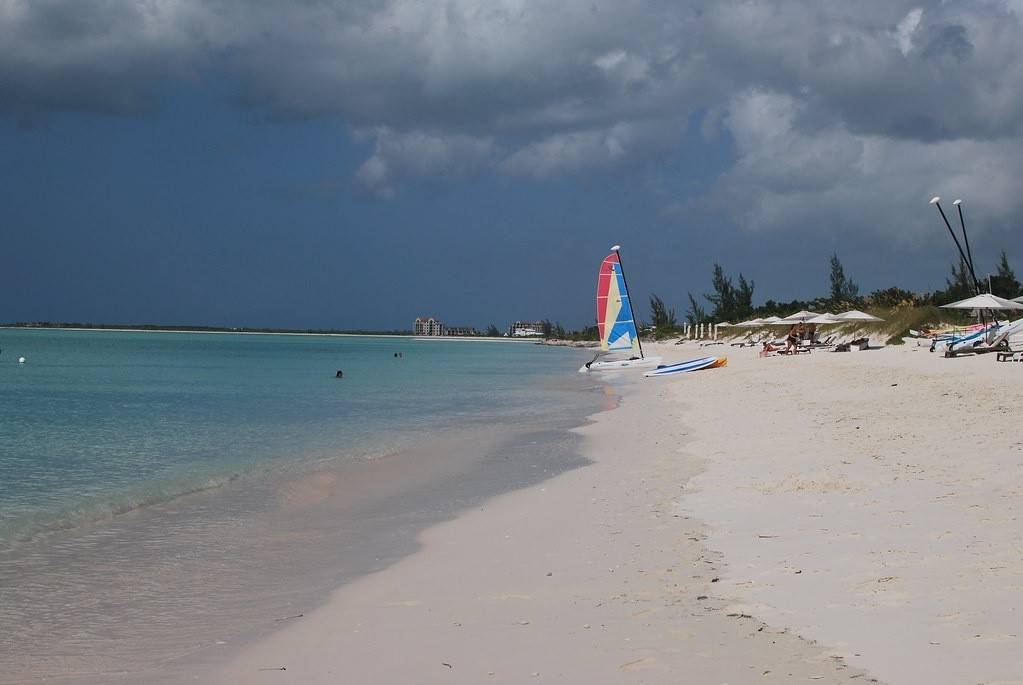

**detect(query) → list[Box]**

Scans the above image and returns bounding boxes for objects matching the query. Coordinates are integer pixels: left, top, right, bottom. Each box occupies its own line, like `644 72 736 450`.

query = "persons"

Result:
785 325 798 355
762 342 780 351
798 322 805 340
336 371 343 378
806 323 816 343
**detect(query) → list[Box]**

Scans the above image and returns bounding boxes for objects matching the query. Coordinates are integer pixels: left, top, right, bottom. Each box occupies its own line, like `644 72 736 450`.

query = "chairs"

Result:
700 327 1023 363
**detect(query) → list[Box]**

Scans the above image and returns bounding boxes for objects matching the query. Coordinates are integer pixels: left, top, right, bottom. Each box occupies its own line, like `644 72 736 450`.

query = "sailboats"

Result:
578 245 662 372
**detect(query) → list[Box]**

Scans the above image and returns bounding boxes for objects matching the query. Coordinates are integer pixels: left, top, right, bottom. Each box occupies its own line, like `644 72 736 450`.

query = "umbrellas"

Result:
745 318 764 341
736 320 764 339
939 294 1023 343
715 322 736 327
826 310 887 341
804 312 846 343
783 310 821 339
755 316 782 336
771 318 806 335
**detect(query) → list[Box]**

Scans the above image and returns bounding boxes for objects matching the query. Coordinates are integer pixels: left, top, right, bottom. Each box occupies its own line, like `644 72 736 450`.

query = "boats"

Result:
902 317 1023 352
642 357 717 377
